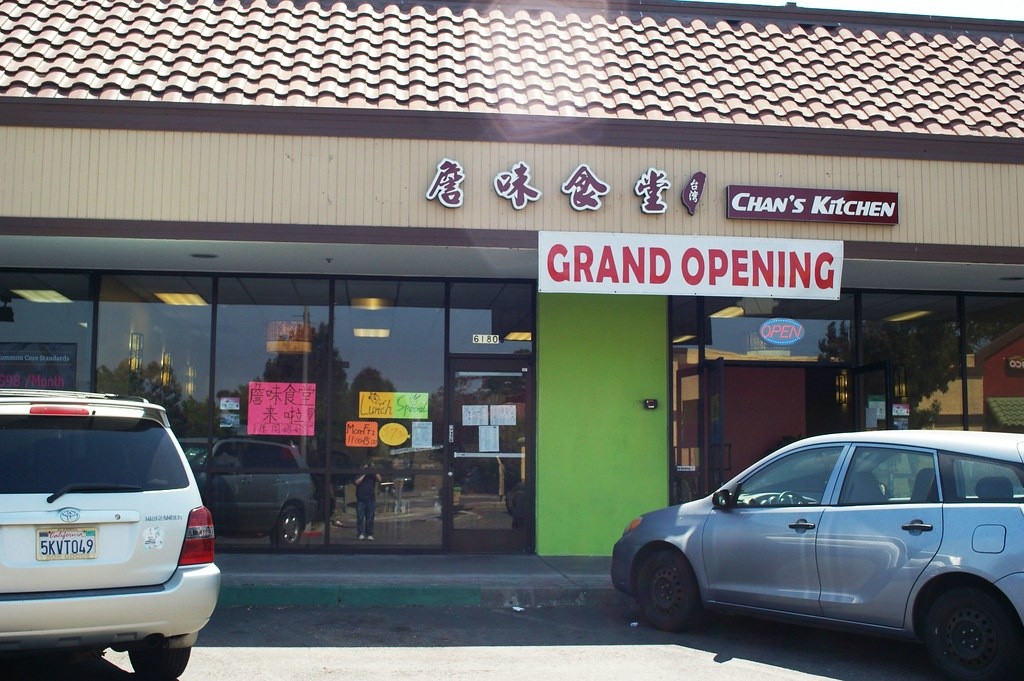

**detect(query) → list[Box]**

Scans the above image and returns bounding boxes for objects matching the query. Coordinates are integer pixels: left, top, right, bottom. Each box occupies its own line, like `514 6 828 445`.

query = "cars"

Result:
610 429 1023 681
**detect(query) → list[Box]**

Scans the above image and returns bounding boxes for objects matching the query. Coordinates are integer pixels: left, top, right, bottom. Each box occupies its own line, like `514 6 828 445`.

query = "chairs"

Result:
976 476 1013 499
845 471 885 504
909 468 942 504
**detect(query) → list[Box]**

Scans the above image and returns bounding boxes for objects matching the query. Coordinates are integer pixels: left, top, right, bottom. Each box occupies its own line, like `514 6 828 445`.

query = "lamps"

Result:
895 364 907 399
264 320 314 355
835 374 848 403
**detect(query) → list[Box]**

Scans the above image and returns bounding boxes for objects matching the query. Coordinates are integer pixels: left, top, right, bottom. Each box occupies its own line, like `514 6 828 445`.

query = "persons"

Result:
435 461 454 521
354 462 381 540
392 453 404 503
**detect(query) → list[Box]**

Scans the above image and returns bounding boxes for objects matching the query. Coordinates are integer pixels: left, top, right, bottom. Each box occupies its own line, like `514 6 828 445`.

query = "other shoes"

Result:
359 535 365 540
368 536 374 540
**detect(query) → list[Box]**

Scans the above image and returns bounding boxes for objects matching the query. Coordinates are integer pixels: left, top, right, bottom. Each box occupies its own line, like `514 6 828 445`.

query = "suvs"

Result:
180 438 315 545
0 387 222 681
